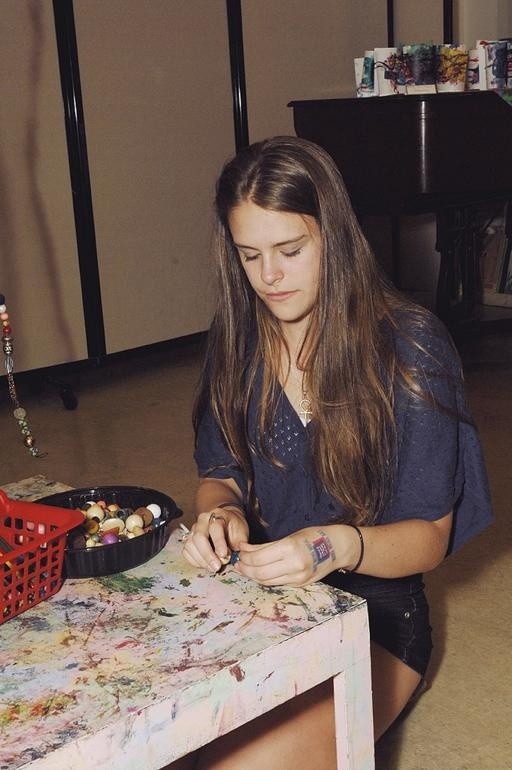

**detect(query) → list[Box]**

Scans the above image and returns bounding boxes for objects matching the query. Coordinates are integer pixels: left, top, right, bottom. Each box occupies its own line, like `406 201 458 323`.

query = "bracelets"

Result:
335 523 364 578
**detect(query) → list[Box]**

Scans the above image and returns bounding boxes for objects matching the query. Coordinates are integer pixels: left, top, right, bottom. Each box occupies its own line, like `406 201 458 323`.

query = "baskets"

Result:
0 490 85 625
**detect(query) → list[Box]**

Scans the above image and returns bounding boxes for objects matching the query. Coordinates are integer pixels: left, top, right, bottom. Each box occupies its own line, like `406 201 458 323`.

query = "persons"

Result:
161 133 495 770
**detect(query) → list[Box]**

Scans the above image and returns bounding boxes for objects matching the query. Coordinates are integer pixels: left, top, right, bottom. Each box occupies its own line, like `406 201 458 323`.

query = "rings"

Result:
180 531 193 543
208 512 227 525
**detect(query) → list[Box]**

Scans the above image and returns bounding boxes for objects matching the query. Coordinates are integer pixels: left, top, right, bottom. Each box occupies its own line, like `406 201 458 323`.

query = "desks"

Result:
0 477 376 770
284 89 512 371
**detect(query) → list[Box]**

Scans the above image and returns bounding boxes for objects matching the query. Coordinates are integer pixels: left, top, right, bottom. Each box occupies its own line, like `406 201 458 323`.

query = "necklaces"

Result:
276 342 320 426
